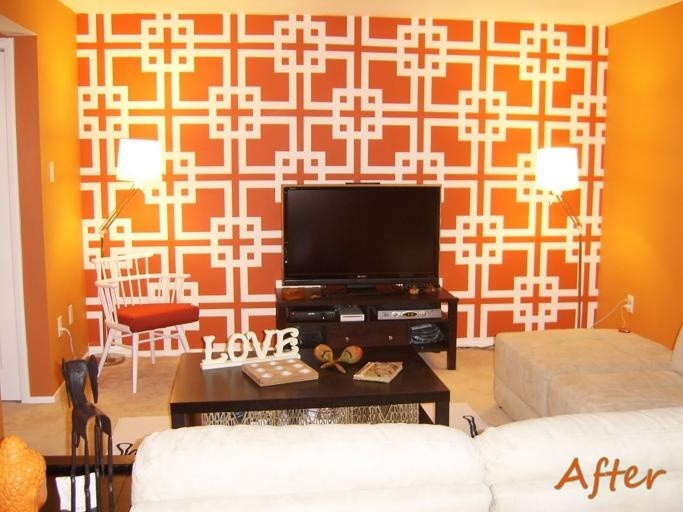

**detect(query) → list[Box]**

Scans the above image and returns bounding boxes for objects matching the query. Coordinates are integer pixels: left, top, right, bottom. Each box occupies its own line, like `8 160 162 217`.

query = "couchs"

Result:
130 408 683 511
491 329 683 415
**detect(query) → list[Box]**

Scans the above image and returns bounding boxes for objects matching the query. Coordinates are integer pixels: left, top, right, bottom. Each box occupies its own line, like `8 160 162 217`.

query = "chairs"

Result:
94 256 198 394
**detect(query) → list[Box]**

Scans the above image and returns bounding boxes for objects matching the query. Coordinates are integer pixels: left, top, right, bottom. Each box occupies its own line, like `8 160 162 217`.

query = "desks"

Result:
273 286 459 370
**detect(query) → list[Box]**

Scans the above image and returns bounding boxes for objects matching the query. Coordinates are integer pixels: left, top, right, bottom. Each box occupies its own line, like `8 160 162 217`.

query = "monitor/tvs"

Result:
279 183 443 298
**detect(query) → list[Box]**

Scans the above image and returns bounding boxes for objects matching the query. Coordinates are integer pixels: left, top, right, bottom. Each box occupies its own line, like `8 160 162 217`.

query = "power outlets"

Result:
623 294 635 312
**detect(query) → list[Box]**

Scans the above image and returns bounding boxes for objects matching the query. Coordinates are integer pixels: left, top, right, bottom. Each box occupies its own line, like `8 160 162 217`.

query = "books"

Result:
353 360 404 384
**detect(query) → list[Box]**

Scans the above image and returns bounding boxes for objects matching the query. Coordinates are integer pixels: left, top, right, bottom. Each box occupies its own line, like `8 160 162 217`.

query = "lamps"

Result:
534 147 584 328
102 138 162 235
